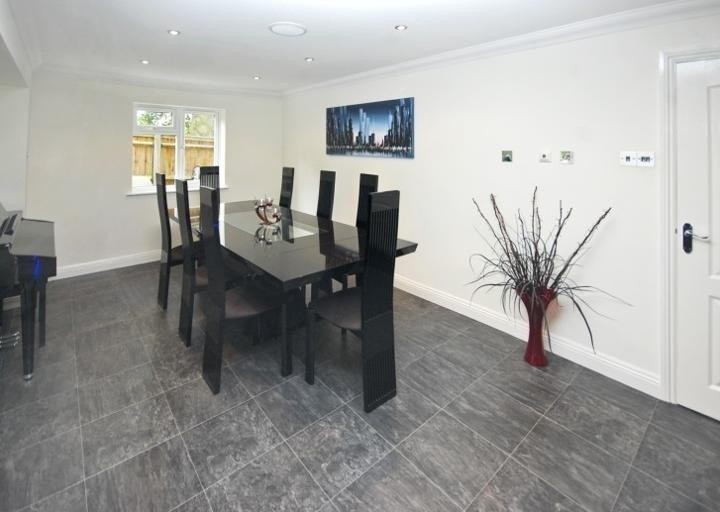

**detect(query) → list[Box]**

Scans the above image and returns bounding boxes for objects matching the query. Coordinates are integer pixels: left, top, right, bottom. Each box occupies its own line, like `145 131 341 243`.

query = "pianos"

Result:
1 205 57 382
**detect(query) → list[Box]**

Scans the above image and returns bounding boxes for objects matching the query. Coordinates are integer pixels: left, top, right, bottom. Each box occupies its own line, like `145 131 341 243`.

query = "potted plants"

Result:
463 185 613 368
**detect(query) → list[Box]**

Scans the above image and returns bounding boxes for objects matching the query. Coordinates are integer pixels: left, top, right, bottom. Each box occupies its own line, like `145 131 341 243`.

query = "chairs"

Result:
304 173 378 337
304 190 400 412
200 166 294 394
278 167 294 208
175 178 259 347
156 172 206 311
316 170 335 219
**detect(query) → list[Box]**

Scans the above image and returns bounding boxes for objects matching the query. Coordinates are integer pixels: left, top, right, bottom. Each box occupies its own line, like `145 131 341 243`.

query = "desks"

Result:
165 199 418 377
